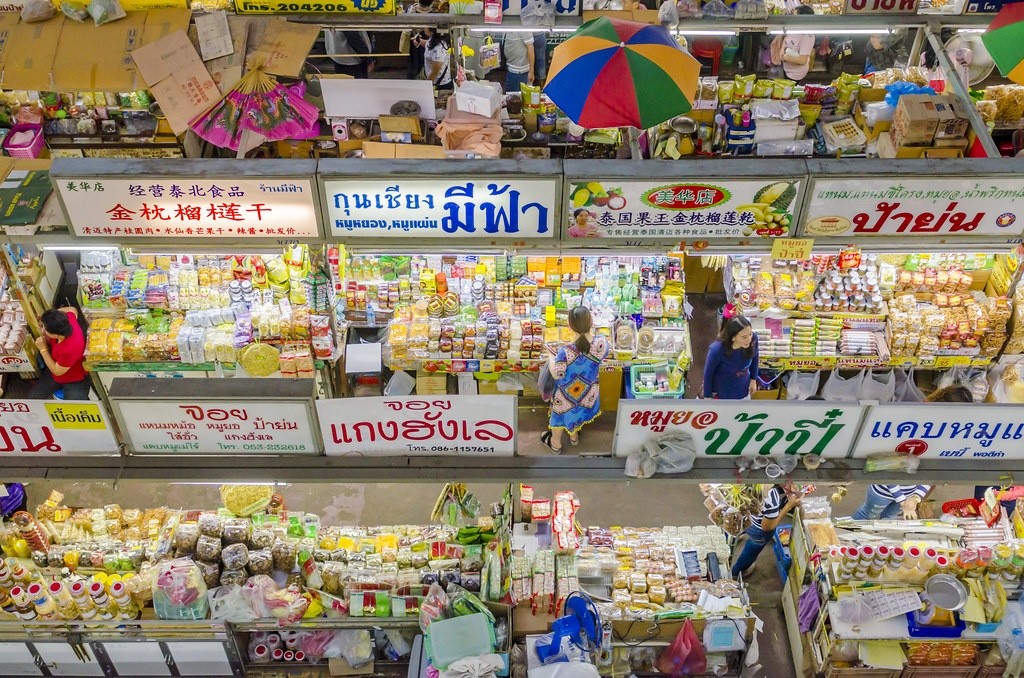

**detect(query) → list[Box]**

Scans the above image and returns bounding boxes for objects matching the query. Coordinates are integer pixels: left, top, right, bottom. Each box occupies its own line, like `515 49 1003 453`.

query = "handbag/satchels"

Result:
696 385 719 400
786 368 821 401
537 356 555 401
399 3 416 53
413 68 437 91
821 365 866 401
860 354 1024 404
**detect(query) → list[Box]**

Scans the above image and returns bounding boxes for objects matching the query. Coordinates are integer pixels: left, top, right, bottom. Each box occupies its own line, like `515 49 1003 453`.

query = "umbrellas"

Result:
543 15 703 132
981 0 1024 88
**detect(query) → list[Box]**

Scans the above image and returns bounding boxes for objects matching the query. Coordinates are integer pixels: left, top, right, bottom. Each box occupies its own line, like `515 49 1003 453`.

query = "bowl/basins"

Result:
670 115 699 134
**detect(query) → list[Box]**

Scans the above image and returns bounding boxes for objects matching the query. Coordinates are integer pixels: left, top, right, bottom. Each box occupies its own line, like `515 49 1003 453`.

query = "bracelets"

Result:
39 349 48 354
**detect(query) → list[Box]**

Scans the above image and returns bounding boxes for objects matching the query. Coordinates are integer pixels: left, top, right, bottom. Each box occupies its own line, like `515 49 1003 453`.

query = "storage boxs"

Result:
0 1 1024 678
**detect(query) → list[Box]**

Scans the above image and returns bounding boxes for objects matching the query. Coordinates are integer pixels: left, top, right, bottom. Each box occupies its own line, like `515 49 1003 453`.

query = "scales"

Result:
147 102 177 142
669 116 699 155
914 574 967 627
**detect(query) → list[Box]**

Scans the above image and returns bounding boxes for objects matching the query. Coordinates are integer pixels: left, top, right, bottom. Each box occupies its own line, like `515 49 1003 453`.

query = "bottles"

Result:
677 104 750 157
366 304 375 327
521 100 584 143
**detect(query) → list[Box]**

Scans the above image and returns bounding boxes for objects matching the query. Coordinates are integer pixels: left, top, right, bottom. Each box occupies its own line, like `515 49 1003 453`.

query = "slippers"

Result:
570 430 580 445
540 431 561 455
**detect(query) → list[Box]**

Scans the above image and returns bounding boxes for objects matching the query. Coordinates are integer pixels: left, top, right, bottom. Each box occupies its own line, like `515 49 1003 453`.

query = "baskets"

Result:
629 364 685 399
3 124 44 160
942 499 981 518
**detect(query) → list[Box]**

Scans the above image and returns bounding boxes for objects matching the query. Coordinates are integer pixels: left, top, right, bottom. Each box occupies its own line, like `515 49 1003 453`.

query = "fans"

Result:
389 101 422 116
940 31 995 90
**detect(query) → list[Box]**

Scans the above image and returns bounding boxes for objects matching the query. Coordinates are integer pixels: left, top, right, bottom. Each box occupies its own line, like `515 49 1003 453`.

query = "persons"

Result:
864 28 939 76
922 384 974 402
703 315 759 400
852 484 935 520
779 6 816 86
36 307 91 401
732 481 805 583
0 483 27 523
325 0 546 97
540 306 609 456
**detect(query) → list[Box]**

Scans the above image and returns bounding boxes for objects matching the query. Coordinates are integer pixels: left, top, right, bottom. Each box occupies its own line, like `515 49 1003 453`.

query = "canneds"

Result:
826 546 950 581
253 631 306 663
229 280 254 303
0 558 140 622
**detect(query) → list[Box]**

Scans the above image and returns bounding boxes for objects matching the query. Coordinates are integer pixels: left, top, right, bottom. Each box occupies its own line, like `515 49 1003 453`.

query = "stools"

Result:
691 37 725 75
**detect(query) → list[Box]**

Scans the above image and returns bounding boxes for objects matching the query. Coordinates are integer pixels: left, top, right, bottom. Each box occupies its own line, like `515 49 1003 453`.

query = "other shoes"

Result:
733 563 755 580
